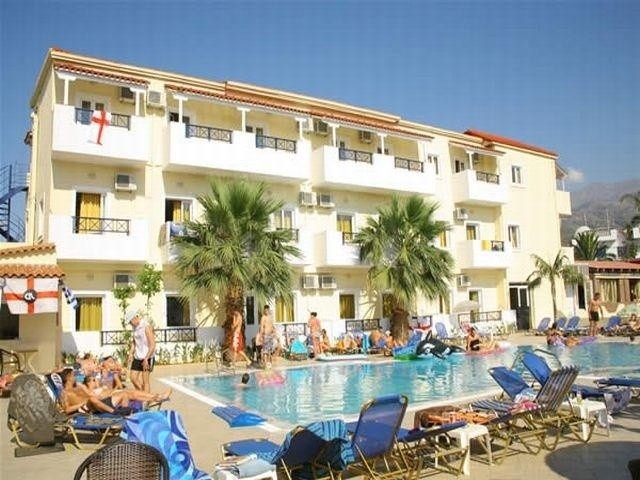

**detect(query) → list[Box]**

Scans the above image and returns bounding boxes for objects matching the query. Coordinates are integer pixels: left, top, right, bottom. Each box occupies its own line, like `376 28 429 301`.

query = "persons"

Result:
546 310 640 348
286 326 395 358
57 352 174 417
127 311 157 391
307 311 321 361
588 291 604 336
259 304 277 367
467 327 480 352
231 307 252 368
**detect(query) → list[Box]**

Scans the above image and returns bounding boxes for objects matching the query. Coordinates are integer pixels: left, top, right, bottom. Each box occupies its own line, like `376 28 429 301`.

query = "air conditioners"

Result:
115 172 138 191
301 274 337 289
300 192 335 208
113 273 138 291
457 275 471 288
454 207 469 220
118 87 135 104
297 117 329 137
471 153 482 163
147 89 164 107
359 130 373 143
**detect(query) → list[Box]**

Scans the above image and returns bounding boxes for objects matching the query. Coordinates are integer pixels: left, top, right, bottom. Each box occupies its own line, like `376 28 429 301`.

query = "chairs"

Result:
286 319 494 361
346 421 468 480
346 393 418 480
9 358 212 480
414 352 640 476
527 316 640 336
221 419 342 480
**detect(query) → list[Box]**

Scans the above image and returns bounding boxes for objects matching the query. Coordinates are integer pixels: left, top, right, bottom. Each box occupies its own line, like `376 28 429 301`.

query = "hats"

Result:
123 310 140 326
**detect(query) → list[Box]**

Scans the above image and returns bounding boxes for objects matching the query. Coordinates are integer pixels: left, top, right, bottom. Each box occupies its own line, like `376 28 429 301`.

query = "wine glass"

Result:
570 393 575 402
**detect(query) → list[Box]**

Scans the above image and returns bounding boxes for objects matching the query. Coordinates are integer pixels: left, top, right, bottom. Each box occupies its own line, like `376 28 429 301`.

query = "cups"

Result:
449 412 458 424
442 412 449 426
575 390 582 405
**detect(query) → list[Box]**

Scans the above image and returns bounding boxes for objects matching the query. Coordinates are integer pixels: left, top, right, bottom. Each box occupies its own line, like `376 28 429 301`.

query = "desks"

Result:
215 459 277 480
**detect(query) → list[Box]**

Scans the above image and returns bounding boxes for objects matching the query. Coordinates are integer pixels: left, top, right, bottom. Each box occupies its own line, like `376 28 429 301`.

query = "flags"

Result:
89 109 112 145
3 278 59 314
59 278 80 311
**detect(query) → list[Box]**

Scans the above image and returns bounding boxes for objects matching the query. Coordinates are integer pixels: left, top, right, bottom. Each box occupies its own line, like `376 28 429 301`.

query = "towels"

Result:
251 420 355 469
